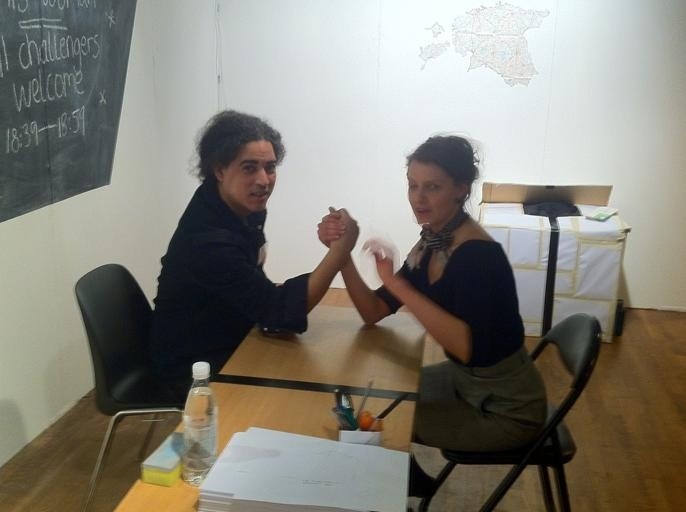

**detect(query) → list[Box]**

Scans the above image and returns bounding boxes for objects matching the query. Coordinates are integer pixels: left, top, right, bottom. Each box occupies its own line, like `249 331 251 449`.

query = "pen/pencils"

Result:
376 393 407 419
355 380 373 421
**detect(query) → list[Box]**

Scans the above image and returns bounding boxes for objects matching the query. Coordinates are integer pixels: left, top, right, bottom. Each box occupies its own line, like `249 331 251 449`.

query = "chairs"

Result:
75 263 184 512
419 313 602 512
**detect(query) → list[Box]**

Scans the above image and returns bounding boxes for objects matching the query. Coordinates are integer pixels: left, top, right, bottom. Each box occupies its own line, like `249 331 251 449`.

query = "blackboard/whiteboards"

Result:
0 0 138 225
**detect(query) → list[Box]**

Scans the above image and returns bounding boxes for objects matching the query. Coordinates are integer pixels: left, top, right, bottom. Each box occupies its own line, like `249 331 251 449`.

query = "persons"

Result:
314 128 549 504
146 107 360 410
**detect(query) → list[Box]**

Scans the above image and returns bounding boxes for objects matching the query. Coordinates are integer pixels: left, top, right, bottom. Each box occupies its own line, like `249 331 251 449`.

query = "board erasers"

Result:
140 432 199 487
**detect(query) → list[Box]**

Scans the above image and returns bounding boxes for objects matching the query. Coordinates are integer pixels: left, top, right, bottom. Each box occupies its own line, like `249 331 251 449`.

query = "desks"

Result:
215 288 426 395
109 378 417 512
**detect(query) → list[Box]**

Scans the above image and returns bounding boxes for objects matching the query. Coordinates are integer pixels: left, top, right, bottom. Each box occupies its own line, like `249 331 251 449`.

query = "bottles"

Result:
181 361 217 487
